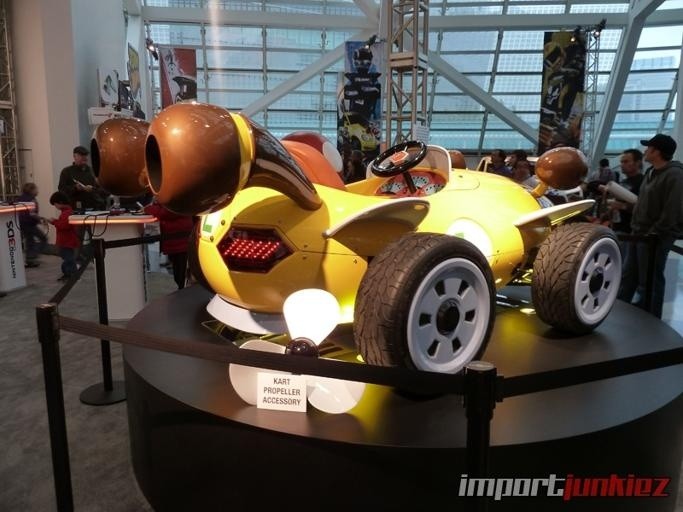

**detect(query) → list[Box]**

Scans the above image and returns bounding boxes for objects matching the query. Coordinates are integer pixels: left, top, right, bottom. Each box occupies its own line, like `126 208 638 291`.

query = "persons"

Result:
619 133 683 319
14 183 47 268
91 100 620 398
58 147 103 270
340 142 618 207
612 148 646 294
47 191 81 283
140 202 194 290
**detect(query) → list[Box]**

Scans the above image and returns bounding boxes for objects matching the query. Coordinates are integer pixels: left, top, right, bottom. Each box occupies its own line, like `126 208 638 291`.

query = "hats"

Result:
640 134 675 155
73 146 89 156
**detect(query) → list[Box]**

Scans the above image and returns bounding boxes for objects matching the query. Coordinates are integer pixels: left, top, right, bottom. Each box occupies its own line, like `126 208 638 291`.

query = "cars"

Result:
476 153 583 202
